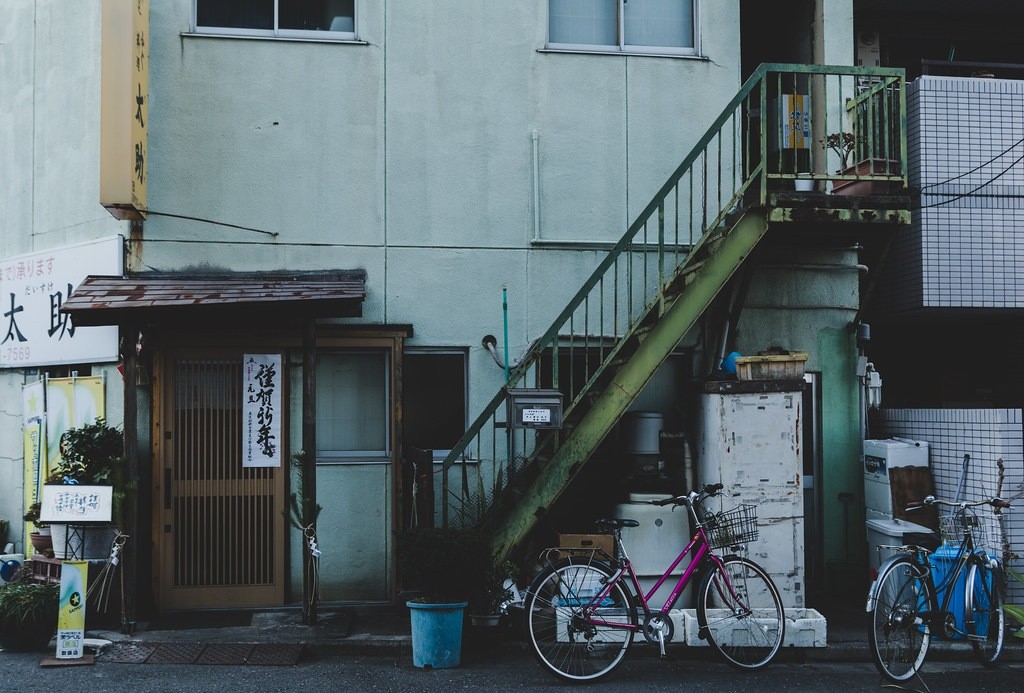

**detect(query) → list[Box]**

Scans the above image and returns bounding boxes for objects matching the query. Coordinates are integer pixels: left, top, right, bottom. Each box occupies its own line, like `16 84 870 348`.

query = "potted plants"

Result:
817 131 901 197
0 577 60 652
23 418 127 561
390 523 521 669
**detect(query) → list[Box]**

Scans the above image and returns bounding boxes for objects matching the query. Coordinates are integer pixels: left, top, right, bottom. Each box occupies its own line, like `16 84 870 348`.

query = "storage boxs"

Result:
573 607 828 647
771 93 810 150
556 530 615 603
733 353 809 379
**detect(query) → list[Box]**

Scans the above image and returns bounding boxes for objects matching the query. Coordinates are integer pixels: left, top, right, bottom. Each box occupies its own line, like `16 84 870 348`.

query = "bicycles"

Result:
866 496 1015 684
524 484 787 682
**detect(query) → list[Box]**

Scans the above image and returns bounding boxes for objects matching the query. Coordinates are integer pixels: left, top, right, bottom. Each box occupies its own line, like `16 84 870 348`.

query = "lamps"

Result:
862 364 883 410
